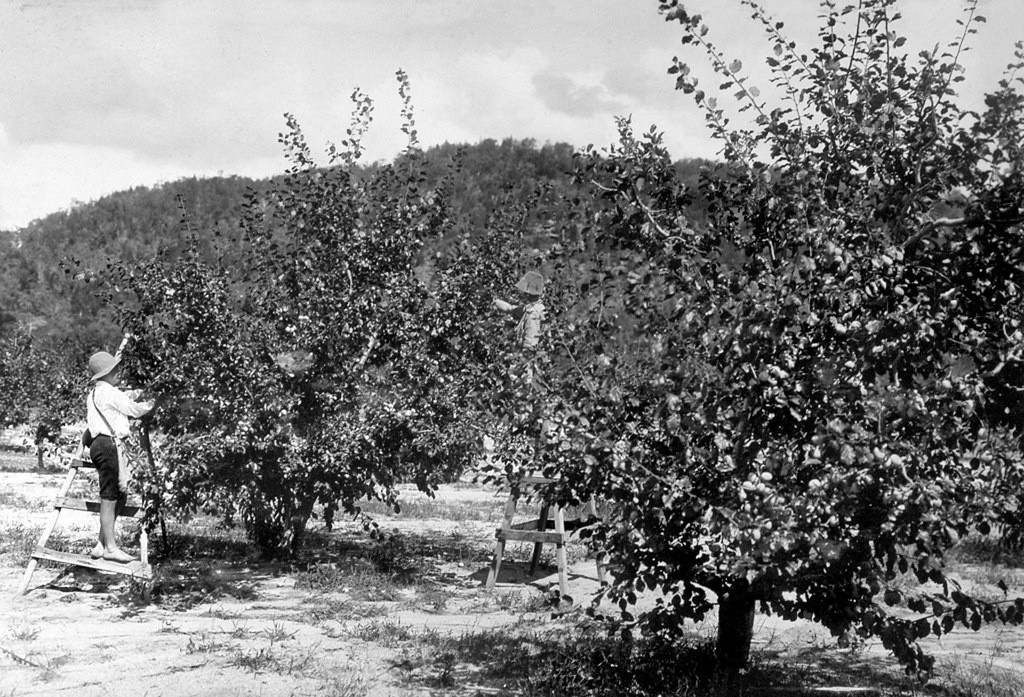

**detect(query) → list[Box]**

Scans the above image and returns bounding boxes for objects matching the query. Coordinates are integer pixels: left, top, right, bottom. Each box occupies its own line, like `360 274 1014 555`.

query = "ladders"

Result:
482 411 606 603
18 419 156 607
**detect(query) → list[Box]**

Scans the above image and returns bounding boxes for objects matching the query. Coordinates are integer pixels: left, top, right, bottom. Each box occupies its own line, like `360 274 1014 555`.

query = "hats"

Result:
88 352 122 382
513 271 544 295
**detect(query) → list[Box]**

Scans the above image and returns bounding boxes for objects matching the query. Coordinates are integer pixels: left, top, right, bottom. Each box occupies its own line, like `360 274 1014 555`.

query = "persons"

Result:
84 351 156 562
492 272 547 353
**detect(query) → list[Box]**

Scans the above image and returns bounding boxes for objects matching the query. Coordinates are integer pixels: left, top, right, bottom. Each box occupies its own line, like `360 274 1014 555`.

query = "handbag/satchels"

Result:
115 438 143 494
